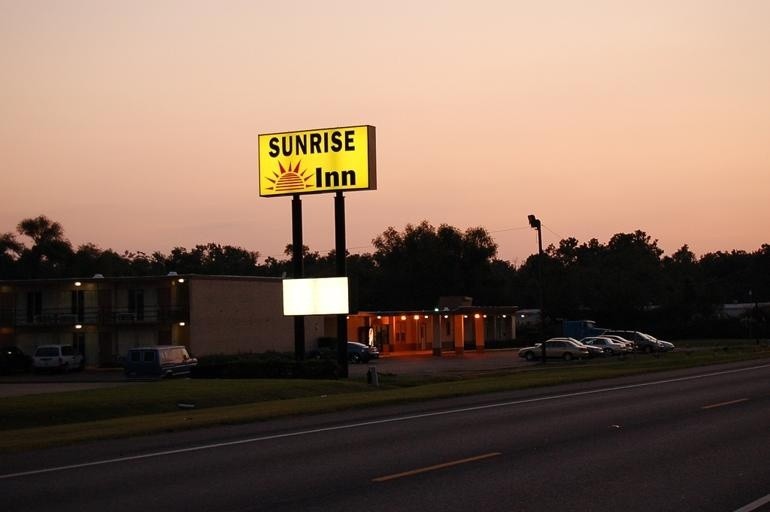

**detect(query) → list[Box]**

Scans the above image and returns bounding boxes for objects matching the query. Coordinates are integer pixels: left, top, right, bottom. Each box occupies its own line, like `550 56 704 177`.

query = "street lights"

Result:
527 214 552 368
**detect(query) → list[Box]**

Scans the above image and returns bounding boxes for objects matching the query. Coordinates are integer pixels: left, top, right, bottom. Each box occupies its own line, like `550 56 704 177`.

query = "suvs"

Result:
33 343 83 371
125 344 201 379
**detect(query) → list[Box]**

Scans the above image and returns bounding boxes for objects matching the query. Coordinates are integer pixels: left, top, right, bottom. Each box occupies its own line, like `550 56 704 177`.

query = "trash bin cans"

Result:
367 366 378 384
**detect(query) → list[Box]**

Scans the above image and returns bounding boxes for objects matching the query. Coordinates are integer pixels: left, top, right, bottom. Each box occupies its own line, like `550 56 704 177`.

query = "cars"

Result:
0 345 35 377
311 336 380 363
519 328 680 360
